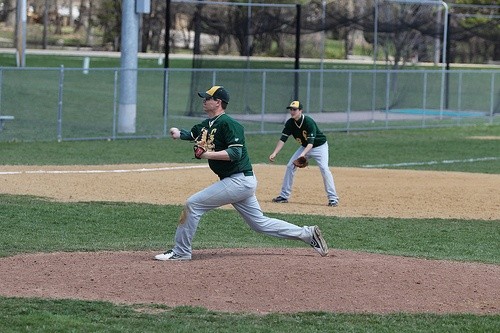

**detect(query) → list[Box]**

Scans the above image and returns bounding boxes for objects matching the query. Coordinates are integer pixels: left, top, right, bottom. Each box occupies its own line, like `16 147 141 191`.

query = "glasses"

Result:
203 97 219 101
290 108 298 111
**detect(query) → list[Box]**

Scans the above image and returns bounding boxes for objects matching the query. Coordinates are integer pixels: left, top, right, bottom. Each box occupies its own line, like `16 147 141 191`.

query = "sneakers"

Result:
271 196 287 203
308 225 329 256
329 200 338 206
155 250 191 260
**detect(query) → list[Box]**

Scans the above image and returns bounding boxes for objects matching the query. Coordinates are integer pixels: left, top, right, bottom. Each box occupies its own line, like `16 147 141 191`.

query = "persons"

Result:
269 101 340 207
154 85 329 261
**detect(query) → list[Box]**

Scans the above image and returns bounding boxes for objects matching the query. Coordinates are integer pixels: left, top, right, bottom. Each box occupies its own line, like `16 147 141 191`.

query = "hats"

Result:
285 101 302 110
197 86 229 104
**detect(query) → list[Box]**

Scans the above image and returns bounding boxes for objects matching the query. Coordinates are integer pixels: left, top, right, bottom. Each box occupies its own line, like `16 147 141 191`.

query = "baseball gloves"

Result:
193 140 209 160
293 156 308 167
